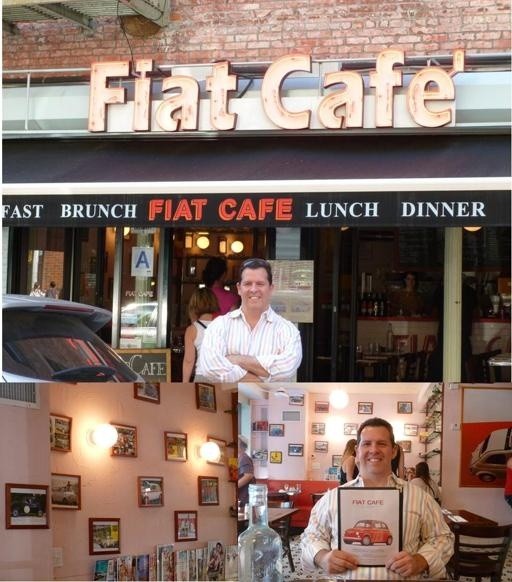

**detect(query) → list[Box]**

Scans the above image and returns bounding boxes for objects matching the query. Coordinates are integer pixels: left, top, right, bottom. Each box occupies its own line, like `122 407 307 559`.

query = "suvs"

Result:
2 292 147 383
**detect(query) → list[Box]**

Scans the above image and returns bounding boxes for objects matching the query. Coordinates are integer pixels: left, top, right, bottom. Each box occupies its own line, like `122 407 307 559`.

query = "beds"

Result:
441 510 497 580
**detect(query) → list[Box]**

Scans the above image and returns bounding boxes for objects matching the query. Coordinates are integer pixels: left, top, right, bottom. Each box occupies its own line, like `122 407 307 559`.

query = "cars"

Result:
5 381 214 550
119 300 158 350
343 519 393 546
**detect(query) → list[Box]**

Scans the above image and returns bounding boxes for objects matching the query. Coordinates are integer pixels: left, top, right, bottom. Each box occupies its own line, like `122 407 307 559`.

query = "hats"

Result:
238 434 248 449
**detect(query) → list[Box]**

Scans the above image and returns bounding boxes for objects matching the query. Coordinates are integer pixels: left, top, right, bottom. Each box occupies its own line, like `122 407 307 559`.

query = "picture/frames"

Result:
248 389 421 569
4 383 237 556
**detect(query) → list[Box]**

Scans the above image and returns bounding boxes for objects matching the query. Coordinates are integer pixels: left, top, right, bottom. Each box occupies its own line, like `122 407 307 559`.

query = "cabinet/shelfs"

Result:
170 227 258 380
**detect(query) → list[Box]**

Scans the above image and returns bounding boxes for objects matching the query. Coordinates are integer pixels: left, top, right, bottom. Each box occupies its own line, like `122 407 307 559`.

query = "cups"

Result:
357 340 379 354
295 483 301 493
284 483 289 490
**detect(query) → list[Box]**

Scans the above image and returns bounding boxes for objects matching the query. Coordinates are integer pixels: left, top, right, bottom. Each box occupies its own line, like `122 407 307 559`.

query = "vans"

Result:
467 427 512 482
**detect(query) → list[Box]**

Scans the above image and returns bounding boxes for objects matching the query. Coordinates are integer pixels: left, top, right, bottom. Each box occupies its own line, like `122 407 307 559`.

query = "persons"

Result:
202 255 242 321
199 257 303 383
182 288 221 383
388 273 424 315
45 281 58 299
405 467 415 481
29 281 44 298
407 461 441 502
238 433 256 508
391 434 405 480
207 542 223 581
340 438 358 485
300 416 456 581
431 268 478 384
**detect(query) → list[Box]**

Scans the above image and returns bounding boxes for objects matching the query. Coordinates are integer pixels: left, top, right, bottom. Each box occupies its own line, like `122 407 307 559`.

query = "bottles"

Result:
361 290 388 317
237 483 283 582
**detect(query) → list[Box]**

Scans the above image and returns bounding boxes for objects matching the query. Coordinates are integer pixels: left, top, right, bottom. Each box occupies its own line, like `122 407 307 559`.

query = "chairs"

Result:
444 521 512 581
321 346 502 383
